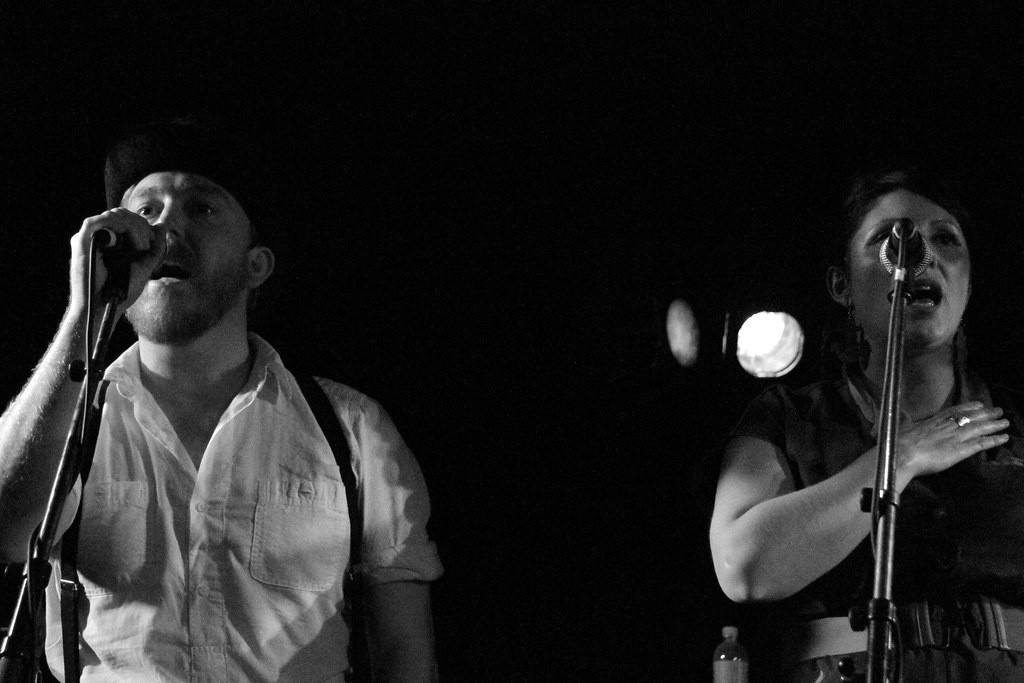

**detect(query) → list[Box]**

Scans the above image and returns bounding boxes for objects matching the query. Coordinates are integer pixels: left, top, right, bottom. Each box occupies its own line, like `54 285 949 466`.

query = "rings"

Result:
945 413 970 426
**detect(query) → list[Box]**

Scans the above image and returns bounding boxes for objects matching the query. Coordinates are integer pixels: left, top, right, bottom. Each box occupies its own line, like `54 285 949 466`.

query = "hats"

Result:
103 113 277 233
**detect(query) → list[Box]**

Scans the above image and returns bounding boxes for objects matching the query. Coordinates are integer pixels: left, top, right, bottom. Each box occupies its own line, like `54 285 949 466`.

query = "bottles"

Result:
713 626 748 683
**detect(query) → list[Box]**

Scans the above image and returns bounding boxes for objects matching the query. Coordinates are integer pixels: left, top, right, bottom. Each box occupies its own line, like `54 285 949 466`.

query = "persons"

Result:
0 110 443 683
709 167 1024 683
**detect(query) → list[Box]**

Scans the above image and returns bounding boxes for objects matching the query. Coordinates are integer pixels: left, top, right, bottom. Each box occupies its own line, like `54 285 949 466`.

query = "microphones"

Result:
93 228 179 264
877 217 933 279
